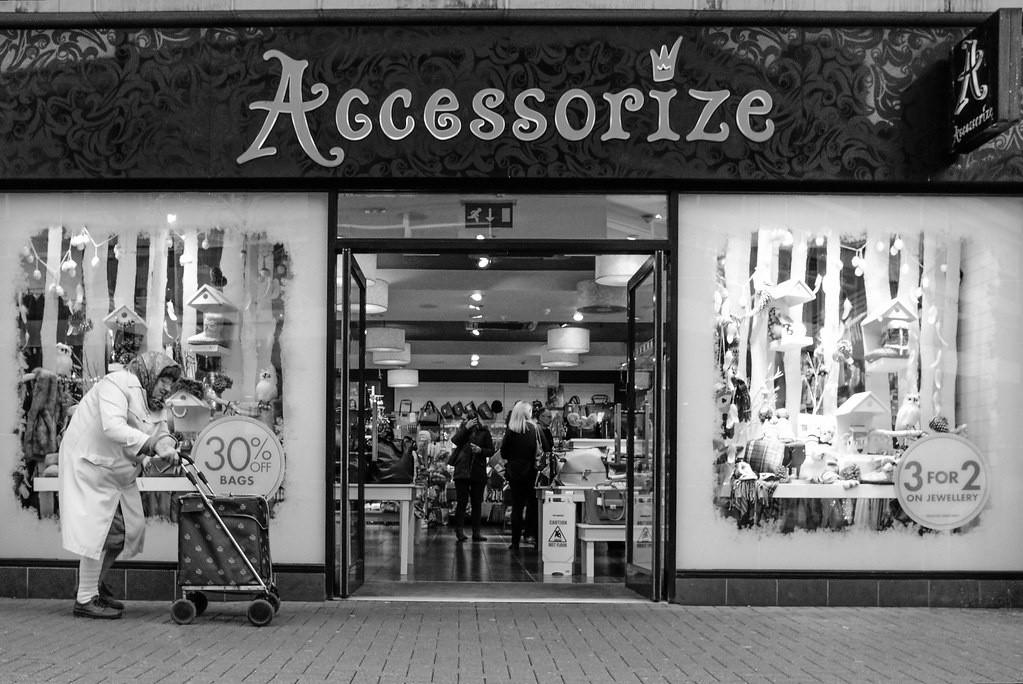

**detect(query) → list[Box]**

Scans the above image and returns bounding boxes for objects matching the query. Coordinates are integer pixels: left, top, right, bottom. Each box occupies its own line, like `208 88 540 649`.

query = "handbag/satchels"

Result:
448 446 472 467
585 395 613 419
534 452 557 490
418 400 441 443
536 427 544 469
585 482 627 526
145 458 182 477
465 401 476 413
453 402 464 416
563 395 585 418
478 401 494 419
554 447 607 486
441 401 454 419
364 436 415 484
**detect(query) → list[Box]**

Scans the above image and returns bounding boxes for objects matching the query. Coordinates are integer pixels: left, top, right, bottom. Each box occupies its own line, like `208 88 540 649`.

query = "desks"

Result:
359 484 424 576
535 436 653 579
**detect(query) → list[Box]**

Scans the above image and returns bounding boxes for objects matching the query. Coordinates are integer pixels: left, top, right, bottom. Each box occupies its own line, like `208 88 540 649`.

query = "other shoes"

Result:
472 533 487 541
456 533 468 540
509 543 519 549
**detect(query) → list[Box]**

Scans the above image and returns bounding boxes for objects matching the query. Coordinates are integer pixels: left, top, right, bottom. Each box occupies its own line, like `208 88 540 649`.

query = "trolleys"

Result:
165 452 281 627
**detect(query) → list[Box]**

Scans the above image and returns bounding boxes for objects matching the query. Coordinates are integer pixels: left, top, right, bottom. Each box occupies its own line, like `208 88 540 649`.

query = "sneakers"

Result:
97 592 124 610
73 595 122 619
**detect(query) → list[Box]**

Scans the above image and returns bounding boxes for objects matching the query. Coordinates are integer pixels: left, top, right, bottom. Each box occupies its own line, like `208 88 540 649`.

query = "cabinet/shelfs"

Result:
408 420 515 524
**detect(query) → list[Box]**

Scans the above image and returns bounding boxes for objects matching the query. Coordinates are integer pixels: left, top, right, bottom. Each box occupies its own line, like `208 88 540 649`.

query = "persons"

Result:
535 407 554 453
447 411 496 542
58 351 183 618
500 399 538 549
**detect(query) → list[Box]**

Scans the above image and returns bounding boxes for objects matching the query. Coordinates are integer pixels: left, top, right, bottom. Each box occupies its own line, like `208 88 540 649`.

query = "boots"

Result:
187 313 232 347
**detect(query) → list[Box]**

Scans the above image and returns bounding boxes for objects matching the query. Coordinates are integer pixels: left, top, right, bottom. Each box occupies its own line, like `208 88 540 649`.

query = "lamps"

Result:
595 254 655 287
577 275 628 312
364 321 406 353
527 370 559 389
540 343 580 367
335 235 377 286
373 343 411 365
547 324 590 355
388 366 418 387
336 276 388 314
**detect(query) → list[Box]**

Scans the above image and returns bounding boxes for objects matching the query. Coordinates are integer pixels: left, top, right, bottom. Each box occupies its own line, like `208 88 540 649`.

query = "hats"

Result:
159 366 180 382
